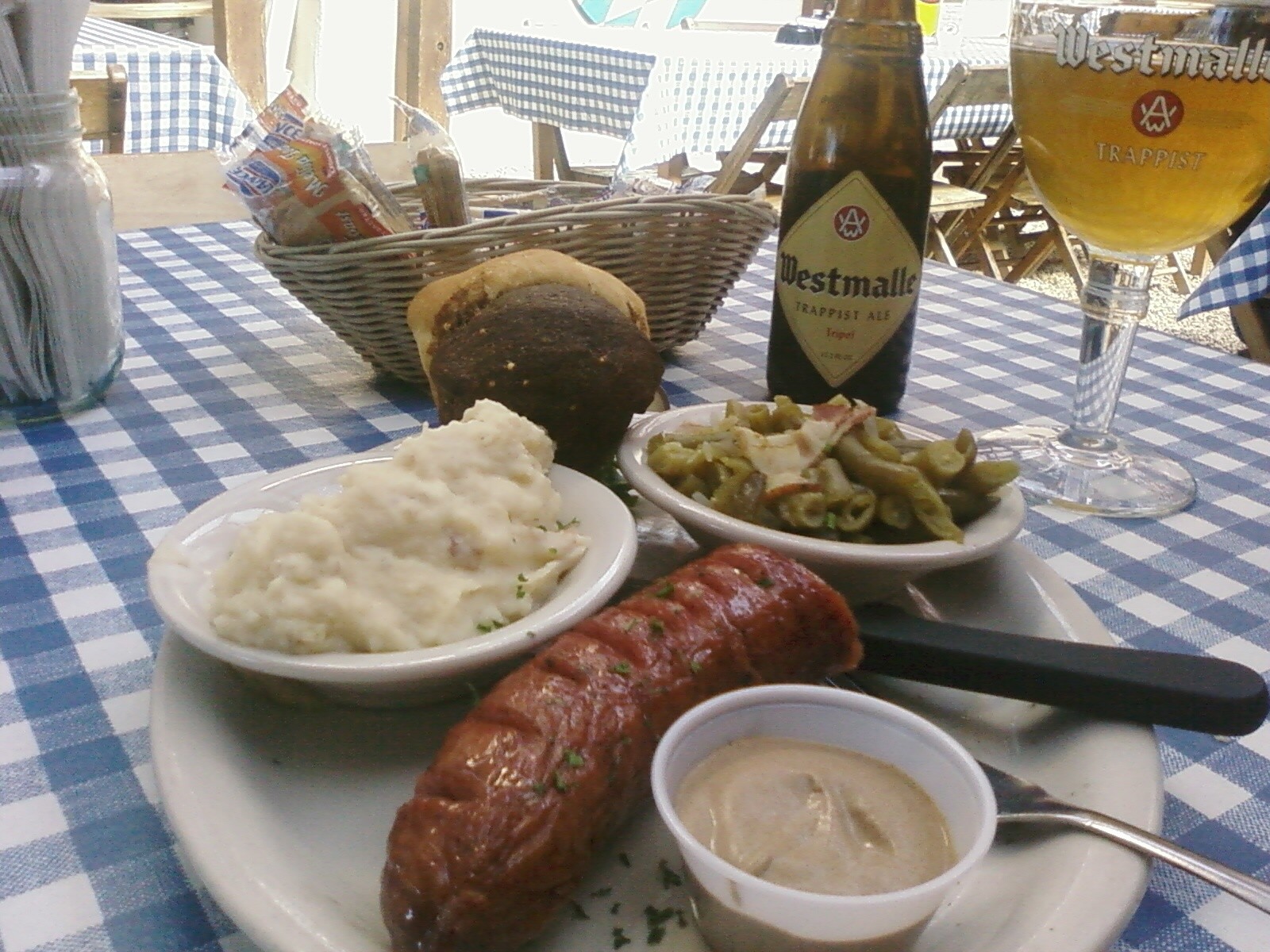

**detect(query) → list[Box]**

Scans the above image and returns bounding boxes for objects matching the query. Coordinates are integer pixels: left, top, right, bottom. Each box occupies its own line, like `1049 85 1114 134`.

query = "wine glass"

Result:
996 0 1270 521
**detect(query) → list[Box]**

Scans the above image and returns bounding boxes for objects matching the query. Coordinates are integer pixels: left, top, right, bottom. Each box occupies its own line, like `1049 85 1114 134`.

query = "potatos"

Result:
212 398 589 656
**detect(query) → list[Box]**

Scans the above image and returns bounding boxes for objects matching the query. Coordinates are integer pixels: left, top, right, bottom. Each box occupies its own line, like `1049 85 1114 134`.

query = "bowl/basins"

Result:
650 684 1001 952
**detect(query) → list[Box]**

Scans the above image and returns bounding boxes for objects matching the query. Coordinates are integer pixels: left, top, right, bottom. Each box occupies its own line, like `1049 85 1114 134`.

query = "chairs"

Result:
68 63 127 151
548 62 1207 312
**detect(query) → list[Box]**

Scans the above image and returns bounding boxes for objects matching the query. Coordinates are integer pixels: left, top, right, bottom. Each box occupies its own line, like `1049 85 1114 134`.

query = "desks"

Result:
439 19 1014 194
69 17 258 155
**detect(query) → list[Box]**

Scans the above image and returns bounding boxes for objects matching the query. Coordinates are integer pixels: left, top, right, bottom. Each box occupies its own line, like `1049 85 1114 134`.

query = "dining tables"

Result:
1 214 1270 952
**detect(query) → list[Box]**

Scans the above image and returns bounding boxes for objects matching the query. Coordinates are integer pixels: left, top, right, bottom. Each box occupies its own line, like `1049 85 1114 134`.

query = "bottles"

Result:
767 0 931 417
1 85 128 427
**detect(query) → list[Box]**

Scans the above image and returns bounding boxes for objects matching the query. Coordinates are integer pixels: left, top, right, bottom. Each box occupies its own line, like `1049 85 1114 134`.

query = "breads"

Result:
407 244 664 478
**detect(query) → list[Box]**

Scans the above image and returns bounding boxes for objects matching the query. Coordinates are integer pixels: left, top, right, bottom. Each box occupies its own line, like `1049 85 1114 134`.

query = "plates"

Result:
148 536 1168 952
618 402 1028 612
144 445 640 715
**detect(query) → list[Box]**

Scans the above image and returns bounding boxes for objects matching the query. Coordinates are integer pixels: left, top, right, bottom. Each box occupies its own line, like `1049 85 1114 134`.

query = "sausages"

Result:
380 537 865 952
